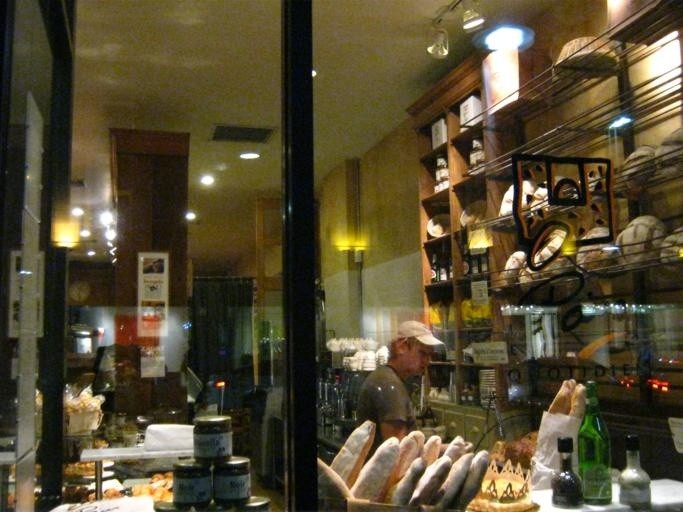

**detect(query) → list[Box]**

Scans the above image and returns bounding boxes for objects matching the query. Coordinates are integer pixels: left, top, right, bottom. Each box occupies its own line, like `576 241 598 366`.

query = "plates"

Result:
459 198 487 227
425 212 450 239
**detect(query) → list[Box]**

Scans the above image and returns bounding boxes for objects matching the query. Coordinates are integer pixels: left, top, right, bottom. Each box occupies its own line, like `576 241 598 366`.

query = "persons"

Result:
357 319 445 449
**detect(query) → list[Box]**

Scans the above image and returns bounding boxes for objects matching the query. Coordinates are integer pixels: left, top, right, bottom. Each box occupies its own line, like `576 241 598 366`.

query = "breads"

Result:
317 379 587 512
7 395 174 504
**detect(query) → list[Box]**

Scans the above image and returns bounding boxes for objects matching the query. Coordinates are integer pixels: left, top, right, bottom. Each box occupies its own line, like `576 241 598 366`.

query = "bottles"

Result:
549 434 584 509
430 243 489 282
616 433 653 511
577 379 613 506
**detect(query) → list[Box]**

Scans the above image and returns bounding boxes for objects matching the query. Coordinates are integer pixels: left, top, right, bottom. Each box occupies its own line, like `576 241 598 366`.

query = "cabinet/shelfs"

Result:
408 1 682 465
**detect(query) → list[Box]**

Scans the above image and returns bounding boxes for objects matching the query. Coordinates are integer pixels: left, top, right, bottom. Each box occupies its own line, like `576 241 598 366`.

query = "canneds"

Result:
153 415 271 512
71 330 92 353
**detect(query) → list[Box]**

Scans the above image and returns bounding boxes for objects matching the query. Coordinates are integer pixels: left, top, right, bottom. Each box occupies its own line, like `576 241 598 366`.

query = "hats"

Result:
398 320 444 346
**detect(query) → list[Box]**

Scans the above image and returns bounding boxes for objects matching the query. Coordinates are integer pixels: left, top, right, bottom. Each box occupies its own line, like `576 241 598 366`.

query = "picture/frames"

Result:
135 249 172 381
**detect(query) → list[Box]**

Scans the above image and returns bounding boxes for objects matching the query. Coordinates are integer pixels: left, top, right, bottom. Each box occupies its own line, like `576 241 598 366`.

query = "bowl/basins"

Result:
477 368 499 412
343 345 390 371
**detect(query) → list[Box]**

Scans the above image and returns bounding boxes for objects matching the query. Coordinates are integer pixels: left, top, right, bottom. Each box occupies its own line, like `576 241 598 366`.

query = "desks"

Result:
80 446 197 501
